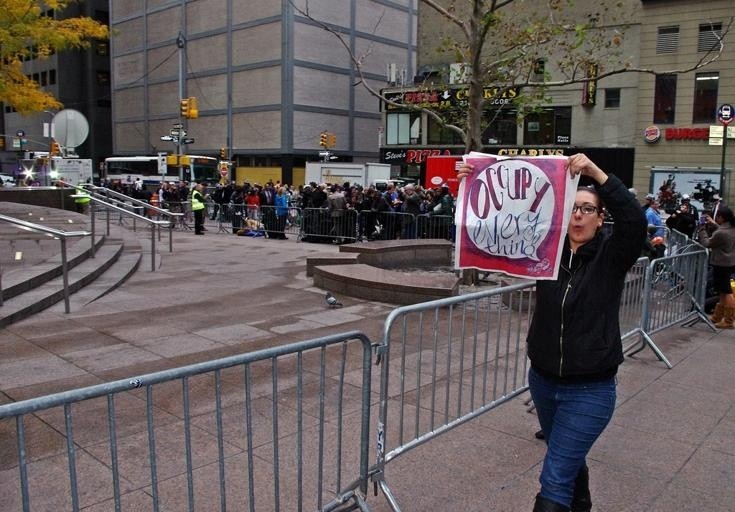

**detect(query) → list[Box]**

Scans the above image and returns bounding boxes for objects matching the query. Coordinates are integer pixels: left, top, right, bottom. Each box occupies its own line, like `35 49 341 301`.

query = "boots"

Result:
709 302 725 323
572 465 592 511
714 304 735 330
529 491 572 512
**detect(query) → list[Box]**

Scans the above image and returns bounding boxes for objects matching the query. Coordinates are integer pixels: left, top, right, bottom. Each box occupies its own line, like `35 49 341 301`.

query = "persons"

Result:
1 169 456 244
598 187 734 330
453 152 648 512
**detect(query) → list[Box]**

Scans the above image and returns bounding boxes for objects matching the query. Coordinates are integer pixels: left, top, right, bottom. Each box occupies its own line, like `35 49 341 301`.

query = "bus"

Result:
104 153 219 195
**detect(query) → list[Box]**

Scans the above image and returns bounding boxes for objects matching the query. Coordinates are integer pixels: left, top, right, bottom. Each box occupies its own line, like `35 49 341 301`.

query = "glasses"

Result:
571 203 602 216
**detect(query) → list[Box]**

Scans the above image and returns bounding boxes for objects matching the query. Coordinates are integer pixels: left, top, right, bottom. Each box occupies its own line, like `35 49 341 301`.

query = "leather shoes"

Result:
195 224 209 235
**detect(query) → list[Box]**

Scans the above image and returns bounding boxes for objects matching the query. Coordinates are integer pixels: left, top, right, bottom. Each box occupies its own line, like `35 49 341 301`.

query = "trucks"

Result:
17 150 95 191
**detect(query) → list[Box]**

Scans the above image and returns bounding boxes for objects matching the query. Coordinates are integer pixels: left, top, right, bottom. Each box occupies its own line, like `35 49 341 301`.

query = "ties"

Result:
267 190 271 203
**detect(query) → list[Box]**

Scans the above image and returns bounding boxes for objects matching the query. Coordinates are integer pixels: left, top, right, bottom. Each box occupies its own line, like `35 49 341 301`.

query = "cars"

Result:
0 173 18 189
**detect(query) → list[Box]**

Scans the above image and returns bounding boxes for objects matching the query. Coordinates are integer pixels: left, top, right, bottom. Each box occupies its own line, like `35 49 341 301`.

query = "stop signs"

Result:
220 167 228 177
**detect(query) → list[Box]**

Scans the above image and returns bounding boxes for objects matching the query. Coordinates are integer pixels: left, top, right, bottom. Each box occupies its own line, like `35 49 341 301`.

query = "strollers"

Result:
228 207 264 237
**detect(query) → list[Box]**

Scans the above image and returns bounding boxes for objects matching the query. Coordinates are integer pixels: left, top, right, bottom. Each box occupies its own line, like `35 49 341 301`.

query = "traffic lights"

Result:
329 134 337 148
220 146 226 160
180 100 190 119
320 132 327 148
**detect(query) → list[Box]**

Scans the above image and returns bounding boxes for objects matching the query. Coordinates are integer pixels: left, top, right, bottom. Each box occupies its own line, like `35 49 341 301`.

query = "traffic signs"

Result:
159 123 196 146
